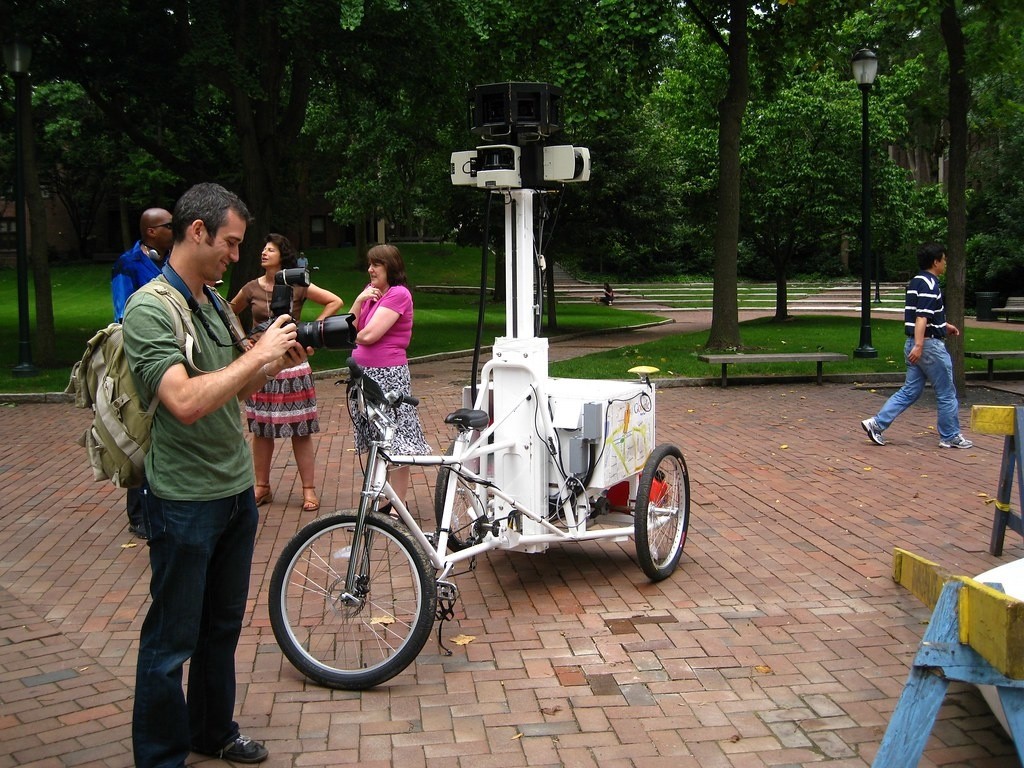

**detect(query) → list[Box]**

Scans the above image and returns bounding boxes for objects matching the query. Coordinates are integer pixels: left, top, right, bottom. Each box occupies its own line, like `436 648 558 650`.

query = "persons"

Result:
298 252 308 268
228 233 344 512
347 244 433 521
860 241 973 449
599 282 614 306
122 183 315 768
110 207 172 540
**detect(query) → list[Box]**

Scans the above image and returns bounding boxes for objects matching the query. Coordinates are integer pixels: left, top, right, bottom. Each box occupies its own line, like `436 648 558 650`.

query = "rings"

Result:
371 291 374 294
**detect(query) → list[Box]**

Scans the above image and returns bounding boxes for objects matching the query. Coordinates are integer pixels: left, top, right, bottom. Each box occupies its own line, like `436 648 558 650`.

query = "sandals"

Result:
302 486 320 511
253 484 273 508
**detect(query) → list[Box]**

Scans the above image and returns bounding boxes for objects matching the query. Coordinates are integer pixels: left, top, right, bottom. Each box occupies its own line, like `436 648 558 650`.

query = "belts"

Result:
909 335 942 340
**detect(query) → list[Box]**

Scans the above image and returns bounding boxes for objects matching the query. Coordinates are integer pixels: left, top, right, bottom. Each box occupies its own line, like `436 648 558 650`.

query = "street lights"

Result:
1 28 44 378
850 47 879 358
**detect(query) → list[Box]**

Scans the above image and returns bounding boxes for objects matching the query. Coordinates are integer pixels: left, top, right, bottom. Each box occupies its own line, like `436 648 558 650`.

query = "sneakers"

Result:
939 433 973 448
860 417 885 445
209 733 269 764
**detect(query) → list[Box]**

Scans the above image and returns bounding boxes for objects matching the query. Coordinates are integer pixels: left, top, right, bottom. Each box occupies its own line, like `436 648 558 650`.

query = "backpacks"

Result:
63 283 244 488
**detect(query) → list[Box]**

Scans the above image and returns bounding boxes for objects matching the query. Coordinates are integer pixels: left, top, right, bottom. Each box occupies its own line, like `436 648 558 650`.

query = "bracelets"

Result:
264 363 274 380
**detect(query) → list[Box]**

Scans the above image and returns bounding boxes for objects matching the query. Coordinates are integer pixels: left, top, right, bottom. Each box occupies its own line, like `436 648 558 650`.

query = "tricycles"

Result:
267 354 691 691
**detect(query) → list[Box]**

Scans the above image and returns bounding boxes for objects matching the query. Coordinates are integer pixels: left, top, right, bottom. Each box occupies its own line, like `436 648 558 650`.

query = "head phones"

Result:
143 242 161 260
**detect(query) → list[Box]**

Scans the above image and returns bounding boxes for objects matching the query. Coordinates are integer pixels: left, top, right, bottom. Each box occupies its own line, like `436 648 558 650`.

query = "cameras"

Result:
270 268 359 350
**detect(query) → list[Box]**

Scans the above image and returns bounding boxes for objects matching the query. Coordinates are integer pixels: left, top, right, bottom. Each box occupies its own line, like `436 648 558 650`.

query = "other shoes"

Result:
128 521 150 540
378 502 392 514
388 502 409 523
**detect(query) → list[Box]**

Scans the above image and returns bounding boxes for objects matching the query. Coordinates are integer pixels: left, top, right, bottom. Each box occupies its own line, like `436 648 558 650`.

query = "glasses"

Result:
149 223 174 230
940 257 948 262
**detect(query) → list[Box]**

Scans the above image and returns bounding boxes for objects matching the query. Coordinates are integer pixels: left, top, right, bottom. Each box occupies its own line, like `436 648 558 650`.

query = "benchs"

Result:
992 297 1024 323
697 353 850 388
965 350 1024 382
